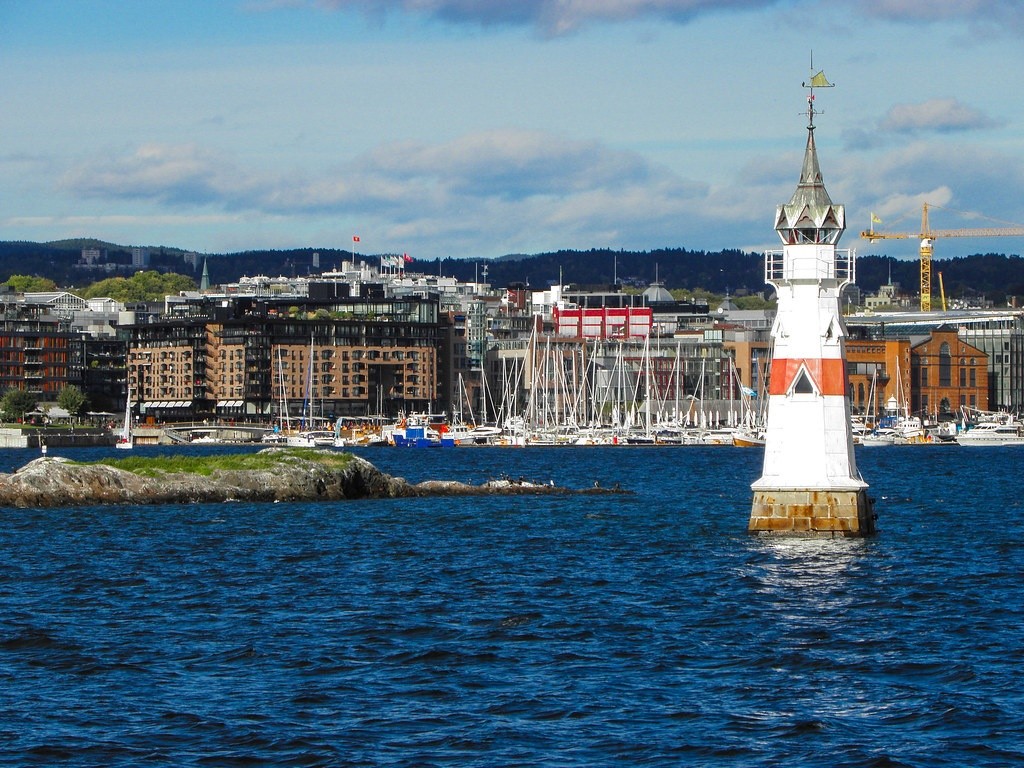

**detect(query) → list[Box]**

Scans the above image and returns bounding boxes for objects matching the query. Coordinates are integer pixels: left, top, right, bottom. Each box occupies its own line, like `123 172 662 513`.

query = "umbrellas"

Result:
87 410 115 424
24 411 43 417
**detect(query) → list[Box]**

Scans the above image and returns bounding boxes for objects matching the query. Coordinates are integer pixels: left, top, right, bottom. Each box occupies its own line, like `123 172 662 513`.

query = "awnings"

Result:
129 401 245 408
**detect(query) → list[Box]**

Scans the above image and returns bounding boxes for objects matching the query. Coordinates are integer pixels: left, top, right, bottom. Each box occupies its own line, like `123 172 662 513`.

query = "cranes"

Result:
859 202 1024 313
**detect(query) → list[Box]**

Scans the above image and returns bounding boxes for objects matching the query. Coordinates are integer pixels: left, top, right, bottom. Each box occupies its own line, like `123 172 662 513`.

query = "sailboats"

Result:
115 380 134 449
261 314 773 446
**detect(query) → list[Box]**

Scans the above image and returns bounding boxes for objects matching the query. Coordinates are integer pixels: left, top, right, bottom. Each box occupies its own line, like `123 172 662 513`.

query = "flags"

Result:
380 252 413 269
354 235 360 242
741 387 756 396
871 213 883 222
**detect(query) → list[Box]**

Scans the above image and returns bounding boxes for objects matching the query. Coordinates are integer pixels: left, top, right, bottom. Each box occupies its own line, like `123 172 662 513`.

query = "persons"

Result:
43 416 49 430
202 418 235 428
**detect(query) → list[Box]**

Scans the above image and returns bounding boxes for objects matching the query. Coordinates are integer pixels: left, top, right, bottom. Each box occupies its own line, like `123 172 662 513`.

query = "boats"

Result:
853 355 1023 446
